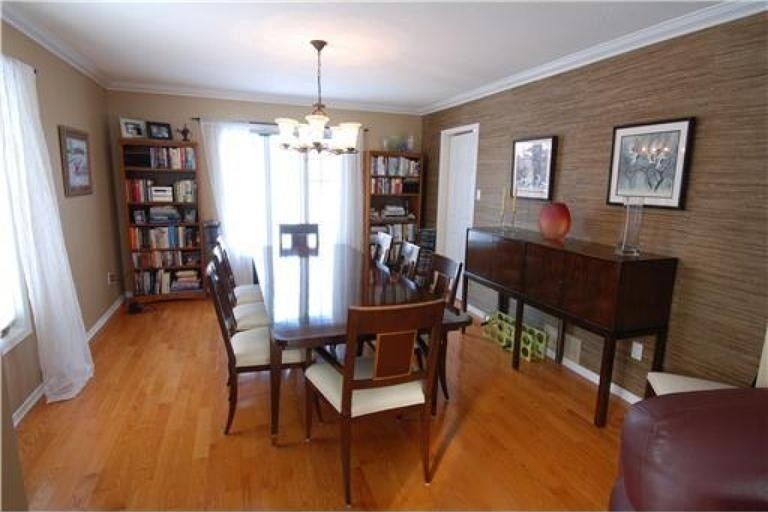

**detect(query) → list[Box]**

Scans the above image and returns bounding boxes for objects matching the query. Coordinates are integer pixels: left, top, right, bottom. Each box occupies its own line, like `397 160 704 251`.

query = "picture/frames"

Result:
510 135 557 202
120 117 147 138
146 121 173 140
606 117 696 210
59 124 93 197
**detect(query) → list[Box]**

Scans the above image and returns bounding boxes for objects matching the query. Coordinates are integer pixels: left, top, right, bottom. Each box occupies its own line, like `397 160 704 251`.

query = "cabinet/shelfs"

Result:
364 149 425 244
117 139 207 302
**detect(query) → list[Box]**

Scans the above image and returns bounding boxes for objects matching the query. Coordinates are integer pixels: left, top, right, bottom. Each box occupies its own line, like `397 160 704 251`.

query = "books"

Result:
131 251 201 271
132 271 202 298
145 146 196 171
370 154 421 178
371 178 404 193
131 206 197 225
368 224 418 267
369 204 409 218
129 226 201 253
126 175 196 202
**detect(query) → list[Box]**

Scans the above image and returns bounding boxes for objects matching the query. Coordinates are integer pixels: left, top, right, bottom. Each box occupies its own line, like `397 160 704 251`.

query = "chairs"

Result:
280 223 319 256
206 260 324 435
358 240 421 356
304 298 446 507
212 243 268 400
417 252 462 400
215 234 263 305
373 230 394 264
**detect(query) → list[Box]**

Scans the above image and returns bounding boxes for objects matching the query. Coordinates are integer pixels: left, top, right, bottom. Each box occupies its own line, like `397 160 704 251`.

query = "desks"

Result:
460 223 679 428
251 243 472 446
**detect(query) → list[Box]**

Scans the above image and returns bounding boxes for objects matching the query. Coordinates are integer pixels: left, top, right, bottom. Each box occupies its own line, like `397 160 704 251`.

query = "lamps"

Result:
274 40 362 157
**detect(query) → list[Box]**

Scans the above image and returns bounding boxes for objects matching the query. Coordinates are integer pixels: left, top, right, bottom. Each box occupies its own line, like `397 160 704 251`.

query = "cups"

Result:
617 194 644 258
